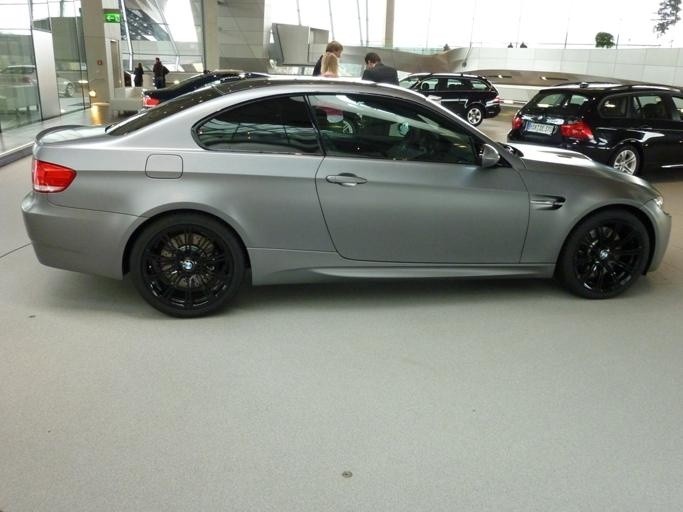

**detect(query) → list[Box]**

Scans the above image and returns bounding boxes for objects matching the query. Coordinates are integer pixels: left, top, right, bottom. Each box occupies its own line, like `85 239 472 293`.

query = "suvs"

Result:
392 73 502 126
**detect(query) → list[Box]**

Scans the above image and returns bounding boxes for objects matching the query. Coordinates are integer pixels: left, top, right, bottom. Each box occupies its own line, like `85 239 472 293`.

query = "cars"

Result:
505 78 682 177
137 70 362 135
3 64 73 97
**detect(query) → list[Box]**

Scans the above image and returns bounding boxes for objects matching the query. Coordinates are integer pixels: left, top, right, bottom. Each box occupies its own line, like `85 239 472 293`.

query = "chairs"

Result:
0 84 38 119
563 100 675 123
422 83 463 92
111 86 158 118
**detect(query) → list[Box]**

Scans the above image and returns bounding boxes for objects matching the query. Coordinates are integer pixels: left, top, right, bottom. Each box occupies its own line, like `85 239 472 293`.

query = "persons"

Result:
319 42 342 77
310 55 325 76
360 52 398 88
133 63 144 87
152 58 163 72
155 62 164 88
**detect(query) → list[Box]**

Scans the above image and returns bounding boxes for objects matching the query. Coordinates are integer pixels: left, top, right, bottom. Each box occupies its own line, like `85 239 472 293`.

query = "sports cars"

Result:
17 73 672 320
